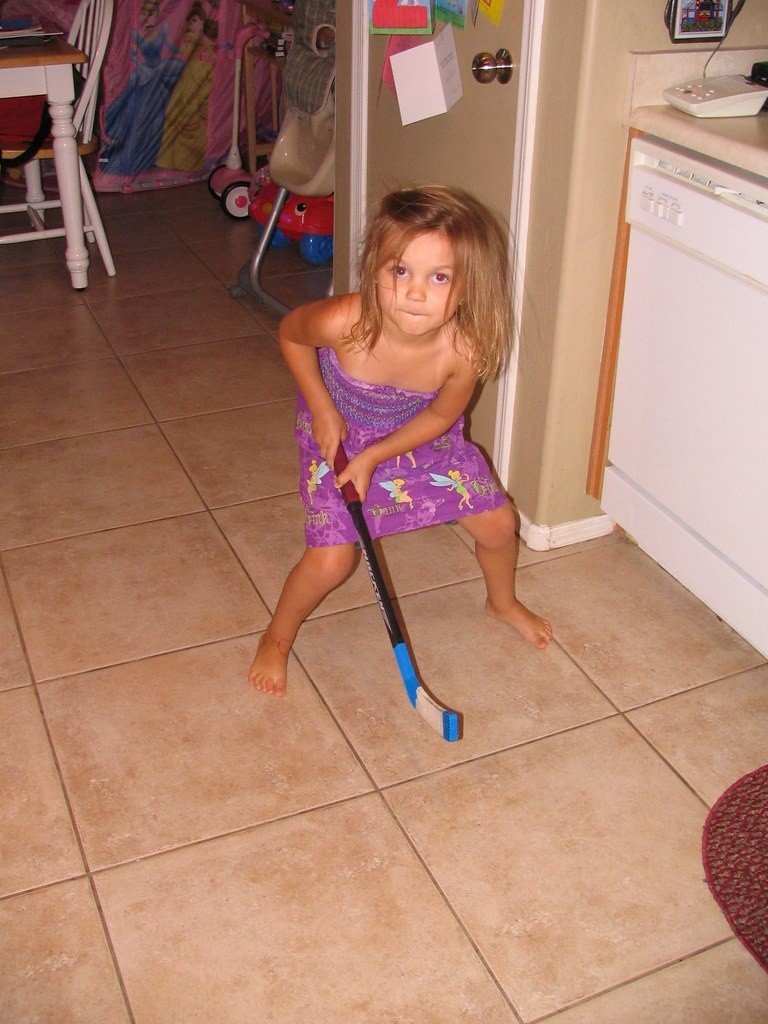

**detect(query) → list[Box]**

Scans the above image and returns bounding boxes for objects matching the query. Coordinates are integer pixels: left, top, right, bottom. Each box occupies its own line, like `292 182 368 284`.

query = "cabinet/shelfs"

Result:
670 0 732 44
597 224 768 664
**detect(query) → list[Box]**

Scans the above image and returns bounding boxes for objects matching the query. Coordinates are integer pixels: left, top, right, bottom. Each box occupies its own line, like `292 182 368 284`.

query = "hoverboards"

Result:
208 22 268 221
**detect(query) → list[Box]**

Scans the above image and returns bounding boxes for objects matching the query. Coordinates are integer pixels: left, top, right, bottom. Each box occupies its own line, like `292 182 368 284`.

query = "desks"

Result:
0 37 91 290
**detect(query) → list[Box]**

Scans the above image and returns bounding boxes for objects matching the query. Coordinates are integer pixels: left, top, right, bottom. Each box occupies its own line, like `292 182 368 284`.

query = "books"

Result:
0 14 64 38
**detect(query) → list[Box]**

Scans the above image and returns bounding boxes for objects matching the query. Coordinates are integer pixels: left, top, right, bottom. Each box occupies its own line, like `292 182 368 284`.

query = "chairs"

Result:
0 0 117 276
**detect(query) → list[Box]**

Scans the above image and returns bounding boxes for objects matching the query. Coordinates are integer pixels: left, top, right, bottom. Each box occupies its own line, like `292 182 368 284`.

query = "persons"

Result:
246 186 554 697
